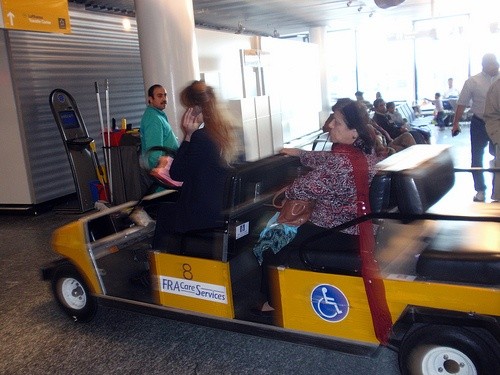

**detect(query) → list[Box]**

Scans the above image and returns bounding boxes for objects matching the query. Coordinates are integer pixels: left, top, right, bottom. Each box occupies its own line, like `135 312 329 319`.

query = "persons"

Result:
253 98 378 312
139 84 180 193
324 90 431 161
452 53 500 201
425 93 446 127
485 76 500 199
153 80 233 235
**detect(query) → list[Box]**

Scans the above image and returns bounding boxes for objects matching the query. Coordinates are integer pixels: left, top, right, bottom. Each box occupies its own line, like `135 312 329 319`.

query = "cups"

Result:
127 124 132 130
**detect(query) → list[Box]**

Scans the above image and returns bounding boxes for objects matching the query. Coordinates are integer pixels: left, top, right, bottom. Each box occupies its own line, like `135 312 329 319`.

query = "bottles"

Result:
112 117 117 130
121 117 126 130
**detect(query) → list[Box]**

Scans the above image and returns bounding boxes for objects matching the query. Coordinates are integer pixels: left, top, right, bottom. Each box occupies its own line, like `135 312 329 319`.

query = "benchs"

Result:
311 100 432 150
162 152 314 263
395 147 500 262
287 144 427 275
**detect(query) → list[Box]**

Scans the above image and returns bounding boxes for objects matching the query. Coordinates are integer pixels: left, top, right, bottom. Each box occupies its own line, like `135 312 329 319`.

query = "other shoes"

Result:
253 304 275 315
473 190 485 200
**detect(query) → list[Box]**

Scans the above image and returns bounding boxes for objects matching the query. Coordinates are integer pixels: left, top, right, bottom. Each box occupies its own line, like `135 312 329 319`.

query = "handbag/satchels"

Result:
273 185 312 226
